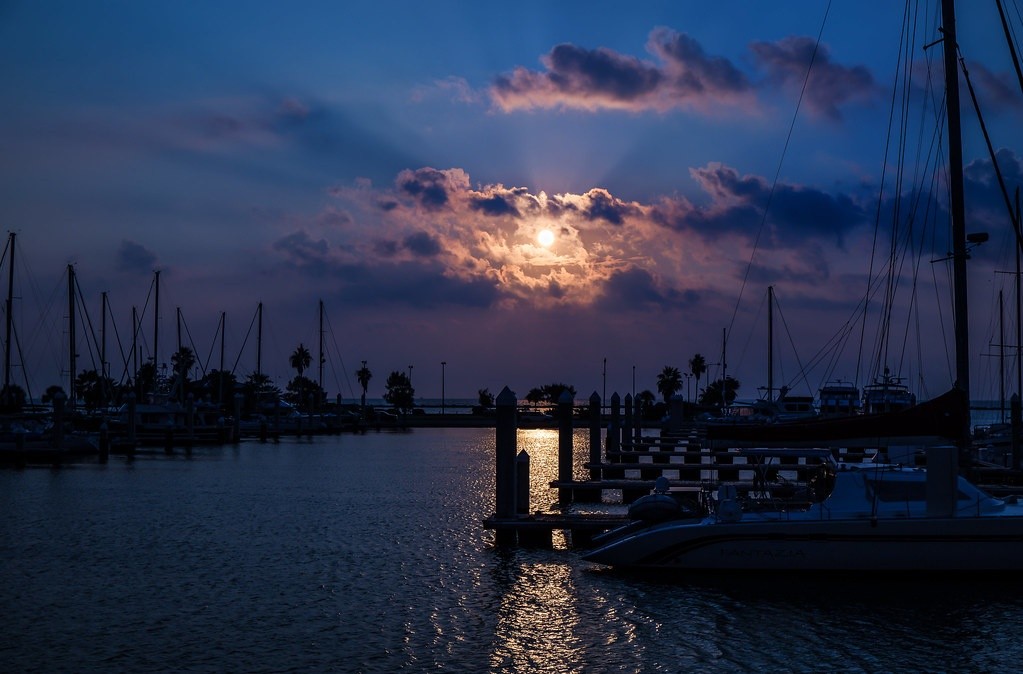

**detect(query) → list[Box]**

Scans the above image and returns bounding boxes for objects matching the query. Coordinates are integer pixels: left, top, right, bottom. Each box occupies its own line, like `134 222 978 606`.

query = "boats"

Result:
816 379 858 416
863 365 915 415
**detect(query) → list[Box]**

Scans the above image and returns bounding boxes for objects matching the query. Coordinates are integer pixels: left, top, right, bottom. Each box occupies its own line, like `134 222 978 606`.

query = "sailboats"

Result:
577 54 1023 589
0 231 374 469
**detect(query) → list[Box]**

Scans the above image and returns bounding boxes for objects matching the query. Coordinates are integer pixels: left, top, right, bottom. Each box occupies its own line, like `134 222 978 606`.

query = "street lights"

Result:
684 372 693 403
706 362 720 388
407 365 413 382
440 361 447 416
602 355 607 414
632 365 635 397
361 359 367 406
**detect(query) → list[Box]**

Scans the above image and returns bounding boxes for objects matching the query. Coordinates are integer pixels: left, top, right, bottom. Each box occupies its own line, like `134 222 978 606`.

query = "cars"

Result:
413 409 426 417
386 409 401 415
379 411 398 422
